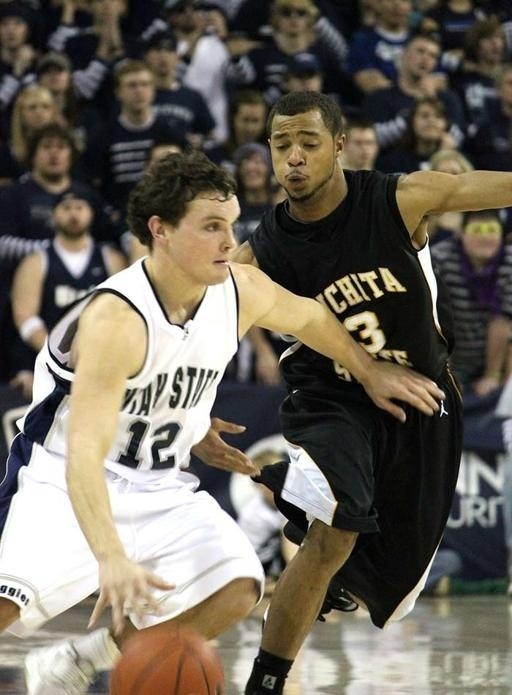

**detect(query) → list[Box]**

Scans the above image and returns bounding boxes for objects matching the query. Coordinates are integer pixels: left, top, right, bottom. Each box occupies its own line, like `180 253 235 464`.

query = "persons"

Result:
194 86 512 695
2 148 446 694
476 315 511 602
234 449 306 619
1 1 511 398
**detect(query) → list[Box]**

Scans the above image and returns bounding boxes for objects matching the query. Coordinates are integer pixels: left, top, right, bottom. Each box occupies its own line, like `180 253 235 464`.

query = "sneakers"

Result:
25 640 99 695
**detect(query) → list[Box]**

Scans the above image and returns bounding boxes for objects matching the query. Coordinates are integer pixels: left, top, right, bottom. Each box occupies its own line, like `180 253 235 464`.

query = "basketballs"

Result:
110 624 226 695
